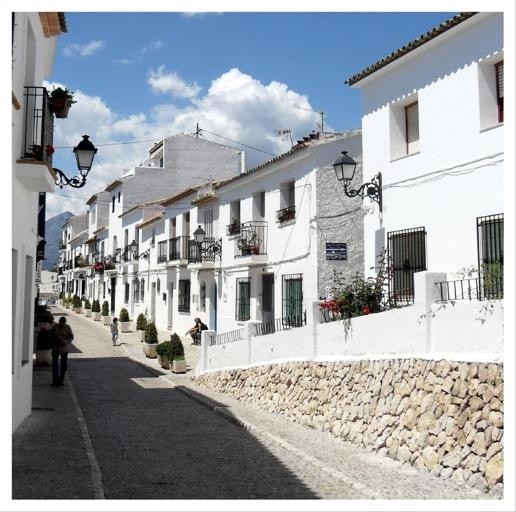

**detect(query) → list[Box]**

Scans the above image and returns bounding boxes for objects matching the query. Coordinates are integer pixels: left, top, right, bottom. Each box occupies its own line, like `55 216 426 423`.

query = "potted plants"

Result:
36 327 52 365
249 229 260 256
60 295 188 374
36 310 54 329
238 238 249 255
47 84 77 119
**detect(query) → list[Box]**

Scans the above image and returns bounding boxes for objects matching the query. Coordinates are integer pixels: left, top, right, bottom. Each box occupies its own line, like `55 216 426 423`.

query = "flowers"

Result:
27 143 54 160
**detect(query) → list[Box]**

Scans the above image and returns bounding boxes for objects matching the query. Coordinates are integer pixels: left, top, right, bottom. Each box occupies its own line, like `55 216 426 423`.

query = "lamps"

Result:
49 134 98 188
129 239 150 259
328 150 387 213
191 227 224 261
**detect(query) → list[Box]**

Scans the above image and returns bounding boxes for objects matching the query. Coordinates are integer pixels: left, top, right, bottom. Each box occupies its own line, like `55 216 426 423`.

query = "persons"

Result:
110 317 119 345
185 318 209 345
46 317 74 387
